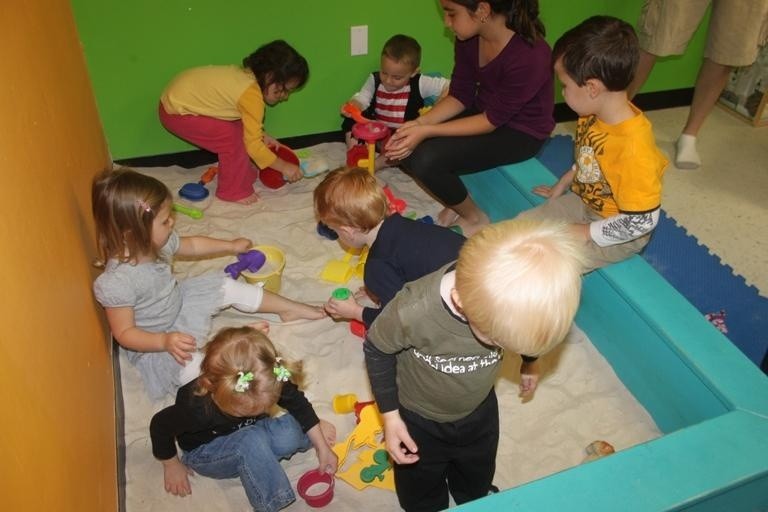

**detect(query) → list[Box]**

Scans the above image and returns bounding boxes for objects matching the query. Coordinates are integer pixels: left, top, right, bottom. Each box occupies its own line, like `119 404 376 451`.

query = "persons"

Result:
515 15 666 279
362 217 589 510
313 165 468 329
341 33 453 157
159 37 310 205
387 4 554 236
625 1 767 172
88 164 332 410
147 323 341 512
